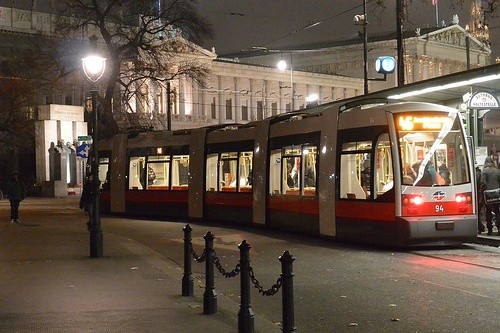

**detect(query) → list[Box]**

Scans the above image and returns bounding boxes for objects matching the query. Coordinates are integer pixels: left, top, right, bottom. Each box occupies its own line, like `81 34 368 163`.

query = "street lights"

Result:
80 34 107 260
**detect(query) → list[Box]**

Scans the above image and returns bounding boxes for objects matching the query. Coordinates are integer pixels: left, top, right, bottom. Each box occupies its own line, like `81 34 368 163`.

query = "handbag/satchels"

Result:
483 188 500 205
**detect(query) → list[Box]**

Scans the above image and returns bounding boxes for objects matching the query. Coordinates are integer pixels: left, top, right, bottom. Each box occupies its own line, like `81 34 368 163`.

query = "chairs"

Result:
240 188 252 192
286 190 299 196
173 186 188 190
303 190 315 196
148 187 168 190
347 193 356 199
222 188 236 191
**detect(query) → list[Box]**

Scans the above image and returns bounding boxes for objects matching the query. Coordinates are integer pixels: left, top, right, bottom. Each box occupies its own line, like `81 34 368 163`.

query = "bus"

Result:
83 95 481 252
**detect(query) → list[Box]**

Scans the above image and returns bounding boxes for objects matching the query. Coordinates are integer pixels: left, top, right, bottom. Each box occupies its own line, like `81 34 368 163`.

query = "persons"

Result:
474 154 500 236
7 171 26 224
82 172 95 231
54 138 94 155
403 160 453 185
285 161 314 188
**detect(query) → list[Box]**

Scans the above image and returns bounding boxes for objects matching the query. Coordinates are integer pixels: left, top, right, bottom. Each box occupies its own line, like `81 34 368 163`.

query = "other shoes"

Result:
10 218 21 224
477 226 486 235
487 229 494 235
497 228 500 236
